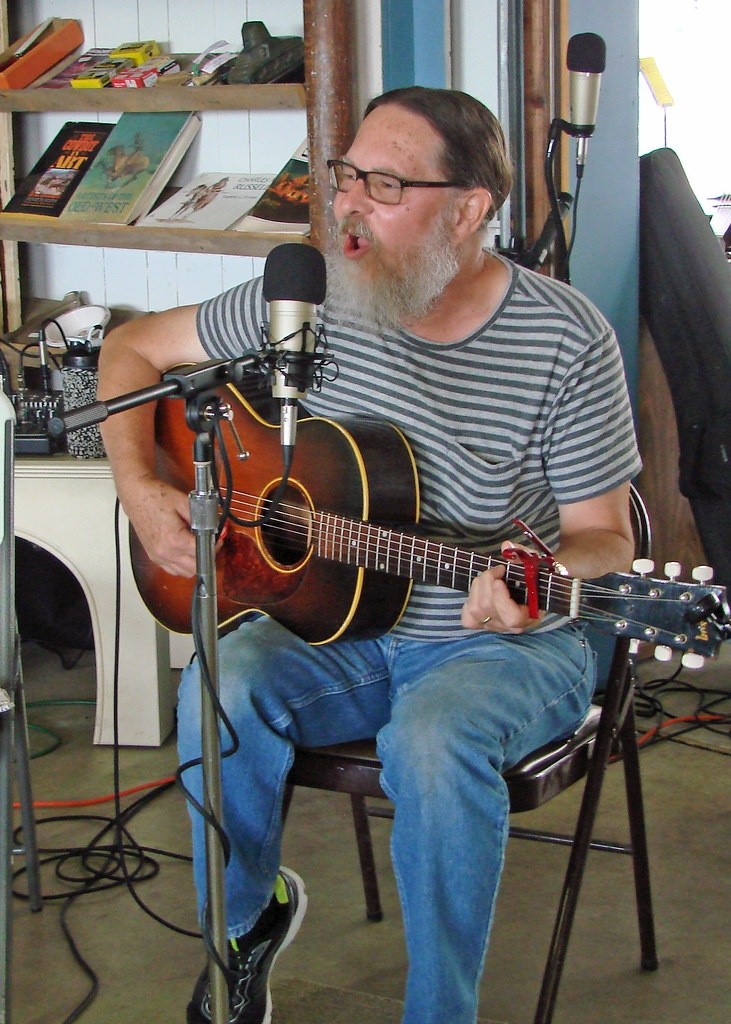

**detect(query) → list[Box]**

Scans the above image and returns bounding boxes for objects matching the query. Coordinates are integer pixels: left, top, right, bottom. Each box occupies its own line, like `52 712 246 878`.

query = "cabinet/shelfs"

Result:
0 0 309 393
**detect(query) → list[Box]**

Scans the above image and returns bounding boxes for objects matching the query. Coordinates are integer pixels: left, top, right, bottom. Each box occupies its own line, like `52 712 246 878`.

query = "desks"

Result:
13 455 174 747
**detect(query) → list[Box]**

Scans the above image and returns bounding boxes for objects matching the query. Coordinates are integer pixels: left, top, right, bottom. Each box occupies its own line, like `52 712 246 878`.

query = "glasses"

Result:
326 158 463 206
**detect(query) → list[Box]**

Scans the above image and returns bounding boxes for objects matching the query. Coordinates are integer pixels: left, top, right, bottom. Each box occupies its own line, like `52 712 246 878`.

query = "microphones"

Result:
568 33 607 177
262 242 327 464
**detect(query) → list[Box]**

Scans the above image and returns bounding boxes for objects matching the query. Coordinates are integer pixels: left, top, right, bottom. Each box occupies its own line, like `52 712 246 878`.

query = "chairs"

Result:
285 479 661 1024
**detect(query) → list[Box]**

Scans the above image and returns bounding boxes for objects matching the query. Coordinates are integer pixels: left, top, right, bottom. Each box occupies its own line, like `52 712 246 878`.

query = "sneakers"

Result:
185 867 308 1024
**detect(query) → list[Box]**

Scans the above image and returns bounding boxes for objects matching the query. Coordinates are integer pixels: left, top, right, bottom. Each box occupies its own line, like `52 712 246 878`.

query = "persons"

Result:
94 88 640 1023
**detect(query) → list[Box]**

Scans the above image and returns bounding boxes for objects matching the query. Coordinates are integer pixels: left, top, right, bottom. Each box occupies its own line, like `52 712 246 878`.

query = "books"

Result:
0 112 312 234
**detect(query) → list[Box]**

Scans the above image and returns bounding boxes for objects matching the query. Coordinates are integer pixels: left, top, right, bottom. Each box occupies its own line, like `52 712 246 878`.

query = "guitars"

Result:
127 363 730 671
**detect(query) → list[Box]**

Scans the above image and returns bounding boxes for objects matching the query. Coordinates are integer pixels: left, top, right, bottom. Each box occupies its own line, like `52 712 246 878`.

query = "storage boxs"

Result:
0 17 84 90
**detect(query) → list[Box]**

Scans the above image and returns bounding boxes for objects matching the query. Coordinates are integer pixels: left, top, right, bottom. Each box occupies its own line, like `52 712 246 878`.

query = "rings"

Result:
479 616 493 626
